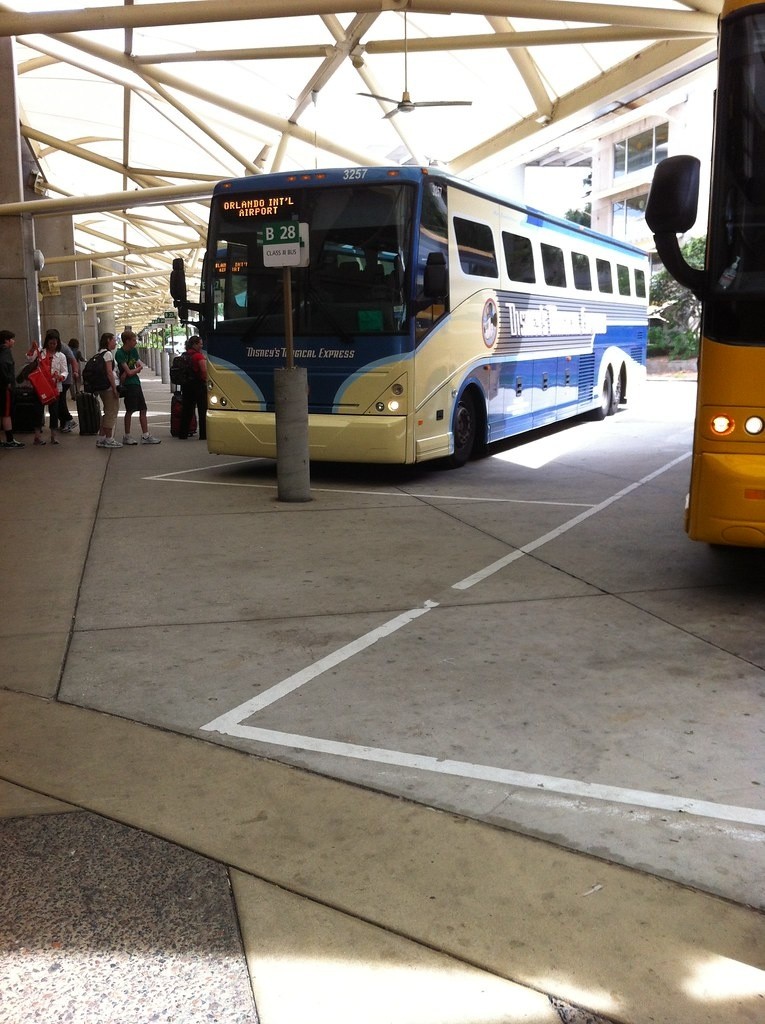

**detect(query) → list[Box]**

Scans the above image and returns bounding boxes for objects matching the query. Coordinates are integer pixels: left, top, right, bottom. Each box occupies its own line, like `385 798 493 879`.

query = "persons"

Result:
45 328 88 433
25 338 68 446
94 331 124 448
180 334 210 441
115 331 162 445
0 328 25 450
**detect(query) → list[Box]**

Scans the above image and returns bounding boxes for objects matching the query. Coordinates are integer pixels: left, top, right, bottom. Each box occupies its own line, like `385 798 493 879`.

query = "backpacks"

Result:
82 351 115 392
170 352 202 391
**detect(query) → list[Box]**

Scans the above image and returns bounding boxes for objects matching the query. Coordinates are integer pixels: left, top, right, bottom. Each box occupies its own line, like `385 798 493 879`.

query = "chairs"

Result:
245 249 448 317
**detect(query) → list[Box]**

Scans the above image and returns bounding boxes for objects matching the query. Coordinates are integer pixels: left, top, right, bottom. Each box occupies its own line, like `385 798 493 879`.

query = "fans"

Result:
355 11 473 121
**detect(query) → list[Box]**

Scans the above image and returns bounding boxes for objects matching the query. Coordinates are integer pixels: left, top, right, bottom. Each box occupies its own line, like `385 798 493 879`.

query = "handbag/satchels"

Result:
28 349 59 405
16 360 38 384
116 385 128 398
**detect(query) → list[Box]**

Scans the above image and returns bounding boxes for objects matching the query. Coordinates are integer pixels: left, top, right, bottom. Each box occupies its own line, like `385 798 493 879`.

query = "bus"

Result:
645 0 765 543
170 167 652 464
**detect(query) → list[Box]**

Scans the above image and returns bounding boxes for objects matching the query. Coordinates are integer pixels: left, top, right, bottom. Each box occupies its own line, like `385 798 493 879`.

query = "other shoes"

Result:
55 427 71 433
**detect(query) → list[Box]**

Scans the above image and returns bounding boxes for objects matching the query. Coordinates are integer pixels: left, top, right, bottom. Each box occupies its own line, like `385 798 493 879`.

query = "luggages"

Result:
11 385 45 434
171 391 197 437
74 374 101 435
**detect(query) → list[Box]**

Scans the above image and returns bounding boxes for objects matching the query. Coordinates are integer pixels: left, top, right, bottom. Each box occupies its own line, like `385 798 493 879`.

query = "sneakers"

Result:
123 435 138 445
33 439 46 446
96 439 123 448
62 419 78 433
51 440 59 444
141 433 161 444
0 439 27 449
